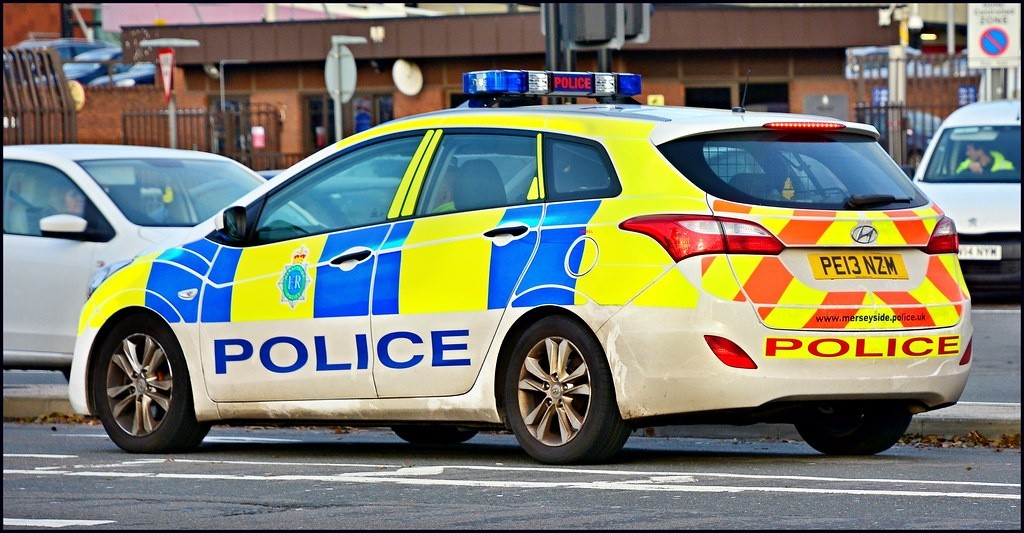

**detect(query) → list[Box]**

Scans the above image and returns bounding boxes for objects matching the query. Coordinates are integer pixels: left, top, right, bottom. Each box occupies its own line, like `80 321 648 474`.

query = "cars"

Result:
2 35 158 92
3 141 324 380
65 70 973 468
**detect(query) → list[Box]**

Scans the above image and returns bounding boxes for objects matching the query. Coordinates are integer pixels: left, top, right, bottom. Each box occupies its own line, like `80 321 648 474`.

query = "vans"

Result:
903 97 1022 302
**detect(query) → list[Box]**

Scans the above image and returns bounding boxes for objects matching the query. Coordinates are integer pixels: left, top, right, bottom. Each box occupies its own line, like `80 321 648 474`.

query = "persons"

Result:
951 139 1015 175
38 179 85 235
426 163 460 218
